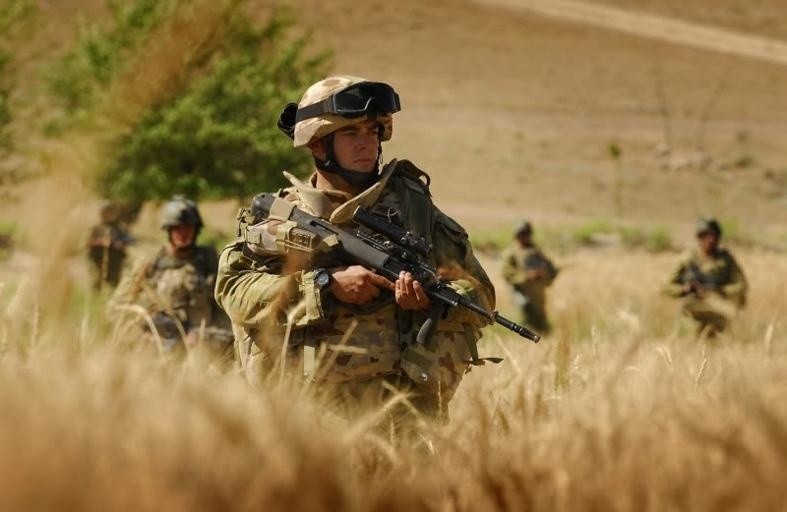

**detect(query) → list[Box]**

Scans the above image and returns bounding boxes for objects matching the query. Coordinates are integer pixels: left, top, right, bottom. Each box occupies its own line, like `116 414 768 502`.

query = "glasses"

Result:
295 81 400 124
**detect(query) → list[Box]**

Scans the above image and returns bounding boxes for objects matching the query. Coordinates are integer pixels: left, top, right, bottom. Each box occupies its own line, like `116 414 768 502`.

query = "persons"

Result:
662 217 749 337
502 221 559 334
107 199 237 375
87 204 126 290
214 74 496 439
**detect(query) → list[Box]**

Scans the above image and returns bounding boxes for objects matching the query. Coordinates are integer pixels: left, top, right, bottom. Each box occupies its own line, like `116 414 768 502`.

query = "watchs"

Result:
314 266 332 308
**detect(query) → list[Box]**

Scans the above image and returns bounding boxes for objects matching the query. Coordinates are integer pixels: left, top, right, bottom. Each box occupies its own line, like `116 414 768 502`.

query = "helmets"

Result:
294 75 393 149
515 223 534 240
160 199 202 230
697 221 720 237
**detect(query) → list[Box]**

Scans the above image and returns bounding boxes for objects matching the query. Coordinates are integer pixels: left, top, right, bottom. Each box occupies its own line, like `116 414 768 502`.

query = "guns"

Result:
142 311 234 344
251 190 541 345
677 262 729 290
